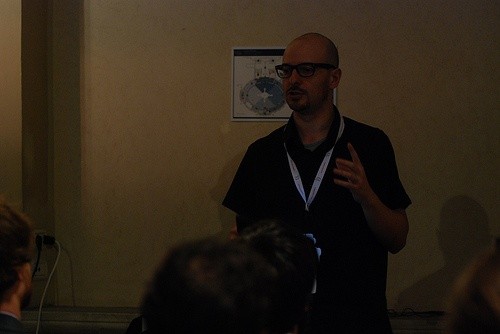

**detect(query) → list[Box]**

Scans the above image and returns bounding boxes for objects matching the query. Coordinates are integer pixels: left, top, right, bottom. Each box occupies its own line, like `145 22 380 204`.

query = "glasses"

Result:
274 62 338 79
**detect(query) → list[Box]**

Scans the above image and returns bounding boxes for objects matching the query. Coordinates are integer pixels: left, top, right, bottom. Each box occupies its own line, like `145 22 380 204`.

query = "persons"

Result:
0 194 35 334
437 238 500 334
140 220 318 334
221 33 413 334
